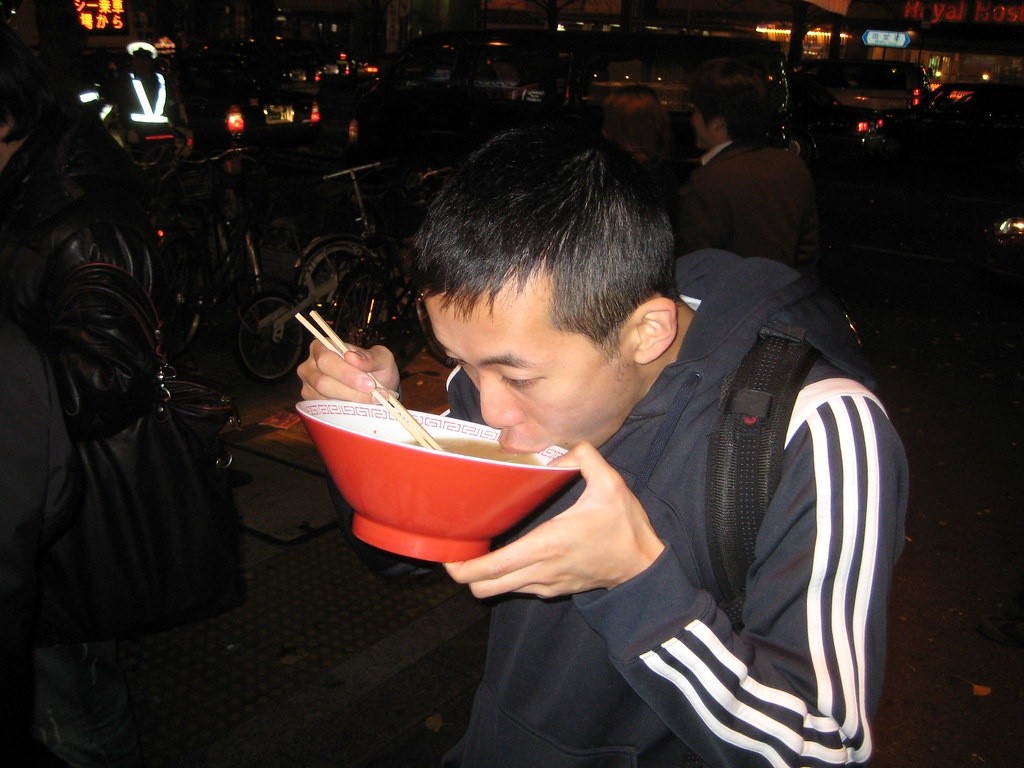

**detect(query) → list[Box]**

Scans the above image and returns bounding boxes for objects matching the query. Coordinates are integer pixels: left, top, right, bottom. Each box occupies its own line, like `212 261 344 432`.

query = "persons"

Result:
0 26 172 768
29 35 844 314
297 118 908 767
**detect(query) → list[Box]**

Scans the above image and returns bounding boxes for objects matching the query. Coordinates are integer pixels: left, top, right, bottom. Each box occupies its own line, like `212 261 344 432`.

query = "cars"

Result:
790 55 1023 295
45 32 381 154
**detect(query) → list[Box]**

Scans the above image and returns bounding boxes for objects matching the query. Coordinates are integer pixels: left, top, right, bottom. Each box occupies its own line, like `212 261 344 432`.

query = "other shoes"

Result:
977 608 1024 649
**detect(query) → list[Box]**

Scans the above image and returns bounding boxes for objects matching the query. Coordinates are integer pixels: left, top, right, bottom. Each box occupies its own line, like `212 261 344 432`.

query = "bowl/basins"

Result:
296 400 581 562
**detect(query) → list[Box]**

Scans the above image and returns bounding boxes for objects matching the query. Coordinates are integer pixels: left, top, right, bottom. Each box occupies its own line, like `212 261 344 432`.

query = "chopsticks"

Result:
295 310 441 451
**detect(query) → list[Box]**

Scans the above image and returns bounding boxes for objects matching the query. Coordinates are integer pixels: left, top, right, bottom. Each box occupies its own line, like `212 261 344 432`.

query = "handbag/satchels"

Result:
2 214 253 648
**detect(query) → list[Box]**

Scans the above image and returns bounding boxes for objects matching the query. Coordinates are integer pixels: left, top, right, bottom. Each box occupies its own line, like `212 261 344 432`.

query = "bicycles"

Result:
121 140 457 384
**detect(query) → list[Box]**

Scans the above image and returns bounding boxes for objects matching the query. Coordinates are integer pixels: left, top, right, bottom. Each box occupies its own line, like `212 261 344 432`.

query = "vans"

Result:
339 26 794 219
